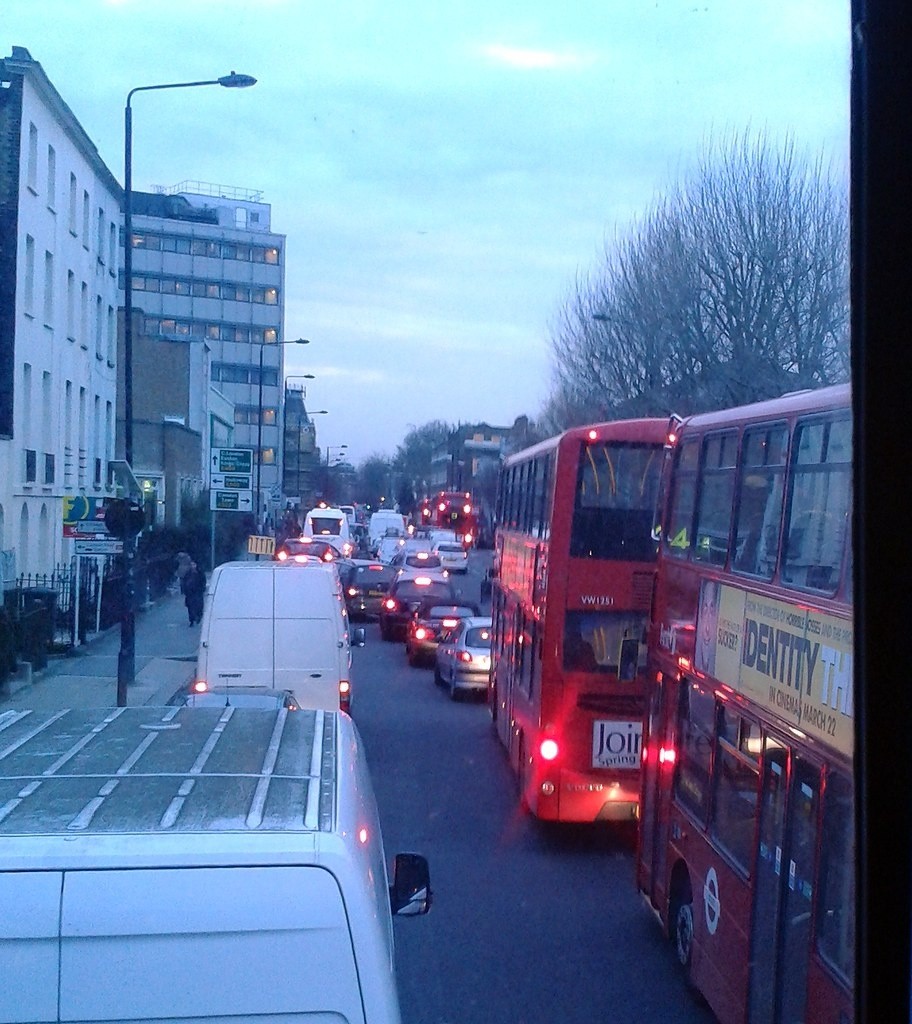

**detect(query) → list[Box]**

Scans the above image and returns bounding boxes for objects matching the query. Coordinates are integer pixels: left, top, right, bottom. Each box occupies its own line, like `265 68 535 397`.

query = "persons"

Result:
265 512 274 533
286 514 301 537
176 549 206 627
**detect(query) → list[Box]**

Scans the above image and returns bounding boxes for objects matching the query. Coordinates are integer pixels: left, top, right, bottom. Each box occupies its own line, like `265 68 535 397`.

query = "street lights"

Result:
295 411 329 512
255 339 310 562
327 445 348 502
119 68 267 684
282 374 315 495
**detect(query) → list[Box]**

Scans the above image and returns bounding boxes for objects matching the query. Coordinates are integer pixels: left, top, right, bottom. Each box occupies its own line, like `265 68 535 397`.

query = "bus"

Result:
625 380 857 1024
476 416 678 823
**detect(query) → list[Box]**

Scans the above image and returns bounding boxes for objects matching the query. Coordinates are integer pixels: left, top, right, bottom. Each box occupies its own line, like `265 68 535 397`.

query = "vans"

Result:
0 699 434 1024
194 557 367 716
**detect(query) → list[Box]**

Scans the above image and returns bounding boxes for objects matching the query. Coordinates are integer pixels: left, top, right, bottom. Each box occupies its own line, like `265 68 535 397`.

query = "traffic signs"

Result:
210 446 254 475
209 489 254 512
209 474 253 491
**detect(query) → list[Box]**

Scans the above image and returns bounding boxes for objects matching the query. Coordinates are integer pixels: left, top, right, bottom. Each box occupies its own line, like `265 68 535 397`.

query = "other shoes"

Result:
197 619 200 624
189 621 194 627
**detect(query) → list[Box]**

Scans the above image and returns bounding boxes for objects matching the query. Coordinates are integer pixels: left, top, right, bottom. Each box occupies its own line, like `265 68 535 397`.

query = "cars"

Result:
432 615 492 702
167 680 301 709
406 597 482 667
273 489 479 641
